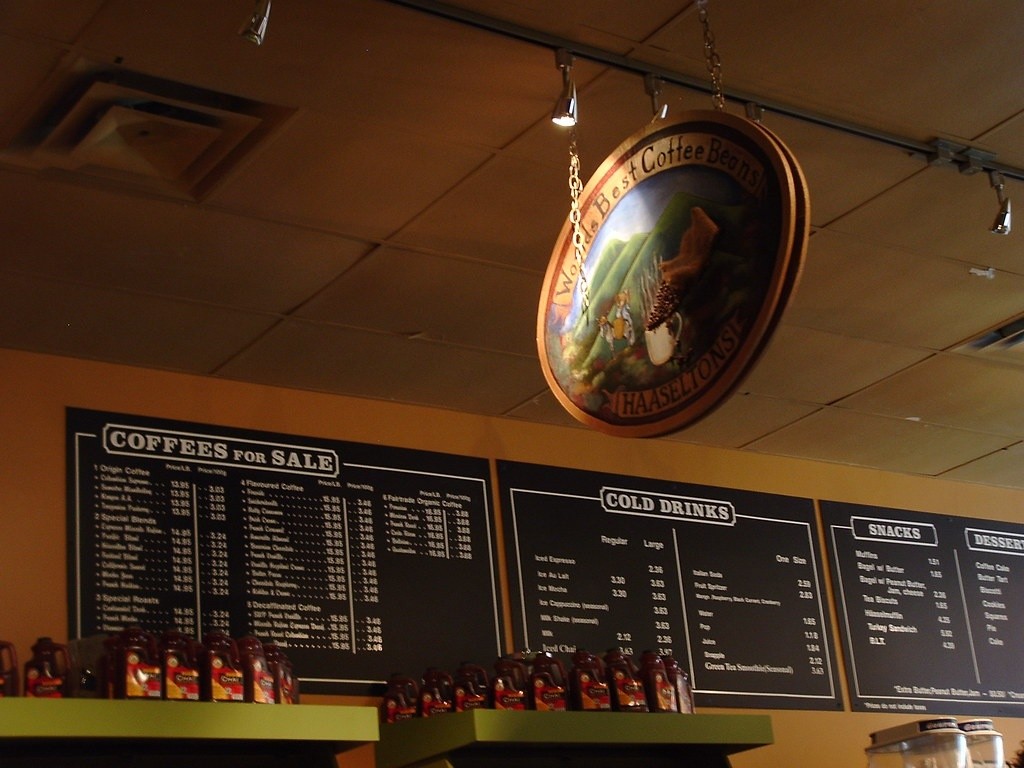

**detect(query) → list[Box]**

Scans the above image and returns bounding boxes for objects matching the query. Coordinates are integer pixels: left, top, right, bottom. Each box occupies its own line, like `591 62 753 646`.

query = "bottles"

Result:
0 621 300 705
379 651 696 724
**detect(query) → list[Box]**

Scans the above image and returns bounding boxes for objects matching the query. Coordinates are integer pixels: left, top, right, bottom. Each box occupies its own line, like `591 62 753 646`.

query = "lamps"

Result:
552 48 578 127
645 74 668 121
989 172 1011 235
237 0 272 46
